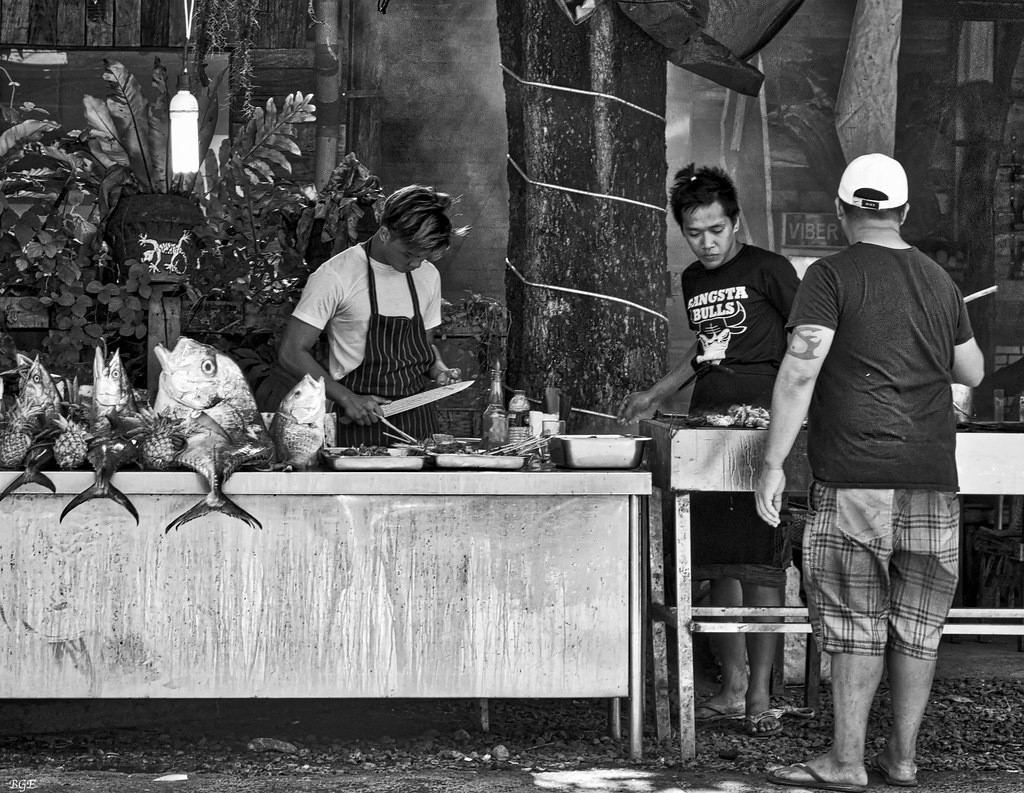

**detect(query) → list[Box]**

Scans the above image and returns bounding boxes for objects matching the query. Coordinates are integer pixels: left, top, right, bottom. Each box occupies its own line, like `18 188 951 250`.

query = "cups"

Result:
542 420 566 461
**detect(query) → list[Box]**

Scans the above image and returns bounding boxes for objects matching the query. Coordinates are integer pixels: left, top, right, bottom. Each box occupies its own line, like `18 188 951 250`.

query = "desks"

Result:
0 429 1024 772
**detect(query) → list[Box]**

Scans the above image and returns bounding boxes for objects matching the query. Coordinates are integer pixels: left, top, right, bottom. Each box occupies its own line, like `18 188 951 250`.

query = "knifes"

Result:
339 380 474 424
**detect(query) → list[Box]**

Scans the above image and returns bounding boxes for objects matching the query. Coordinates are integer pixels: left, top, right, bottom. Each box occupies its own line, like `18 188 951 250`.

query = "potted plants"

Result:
0 60 323 290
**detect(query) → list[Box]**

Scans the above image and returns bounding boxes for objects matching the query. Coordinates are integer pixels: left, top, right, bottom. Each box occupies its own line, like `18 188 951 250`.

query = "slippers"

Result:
768 762 867 792
872 750 918 787
745 711 784 737
693 702 746 722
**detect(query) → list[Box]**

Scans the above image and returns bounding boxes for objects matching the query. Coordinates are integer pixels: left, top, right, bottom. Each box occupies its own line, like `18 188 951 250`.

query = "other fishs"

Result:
1 336 326 532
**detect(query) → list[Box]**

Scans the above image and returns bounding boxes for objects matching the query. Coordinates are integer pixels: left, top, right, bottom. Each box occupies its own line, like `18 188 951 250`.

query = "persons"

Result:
754 154 984 791
279 184 463 449
617 163 802 737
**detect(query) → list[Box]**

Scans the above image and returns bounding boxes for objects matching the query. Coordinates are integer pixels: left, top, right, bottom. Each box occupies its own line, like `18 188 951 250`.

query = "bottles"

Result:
483 370 508 450
508 390 531 444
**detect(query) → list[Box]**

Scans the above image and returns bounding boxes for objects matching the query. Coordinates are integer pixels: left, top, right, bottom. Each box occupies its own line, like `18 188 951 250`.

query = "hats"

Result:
839 152 908 210
